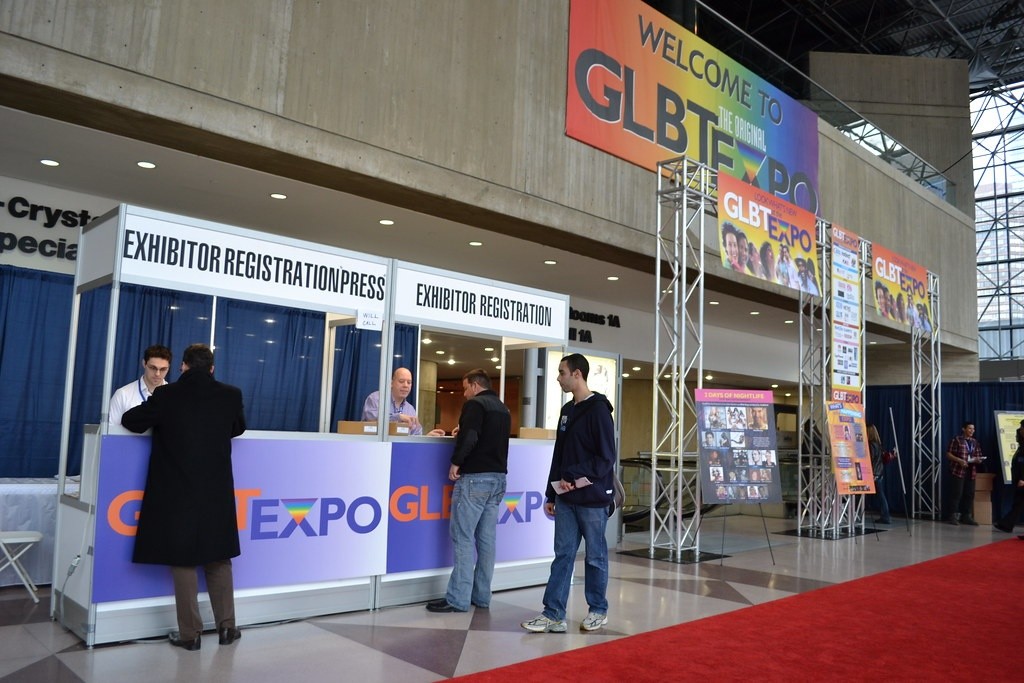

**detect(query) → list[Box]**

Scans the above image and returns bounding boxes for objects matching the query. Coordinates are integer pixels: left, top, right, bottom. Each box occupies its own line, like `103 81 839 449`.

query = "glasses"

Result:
146 364 169 373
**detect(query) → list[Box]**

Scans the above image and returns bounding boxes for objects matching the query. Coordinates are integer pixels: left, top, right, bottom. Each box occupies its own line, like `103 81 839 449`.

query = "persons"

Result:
109 345 172 425
121 344 246 651
522 353 616 632
875 281 932 331
704 406 775 498
945 422 985 526
791 419 827 499
722 220 820 295
856 424 891 524
362 367 445 437
994 420 1024 533
426 369 512 608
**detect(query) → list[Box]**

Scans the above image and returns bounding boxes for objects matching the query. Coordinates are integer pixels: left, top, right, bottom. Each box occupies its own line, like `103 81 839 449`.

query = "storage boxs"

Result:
974 490 991 502
974 512 992 525
974 473 996 491
974 502 992 513
518 428 556 439
389 422 409 435
338 421 379 435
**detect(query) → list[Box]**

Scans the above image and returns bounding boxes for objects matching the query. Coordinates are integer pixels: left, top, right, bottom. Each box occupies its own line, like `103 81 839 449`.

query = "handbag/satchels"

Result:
608 470 625 518
881 447 894 464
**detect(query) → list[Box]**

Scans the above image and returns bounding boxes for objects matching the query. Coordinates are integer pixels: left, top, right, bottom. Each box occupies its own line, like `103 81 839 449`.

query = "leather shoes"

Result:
218 626 242 645
168 631 201 651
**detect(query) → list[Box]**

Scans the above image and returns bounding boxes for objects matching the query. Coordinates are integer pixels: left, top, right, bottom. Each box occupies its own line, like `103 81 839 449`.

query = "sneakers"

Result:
520 615 567 633
426 598 463 613
579 612 609 631
875 519 891 525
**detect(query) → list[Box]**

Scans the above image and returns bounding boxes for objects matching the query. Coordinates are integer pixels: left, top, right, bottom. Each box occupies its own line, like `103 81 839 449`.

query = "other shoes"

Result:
963 520 979 525
994 524 1014 533
949 519 959 525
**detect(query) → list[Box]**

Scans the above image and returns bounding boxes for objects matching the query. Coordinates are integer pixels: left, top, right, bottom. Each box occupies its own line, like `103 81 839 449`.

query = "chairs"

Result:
0 531 43 603
